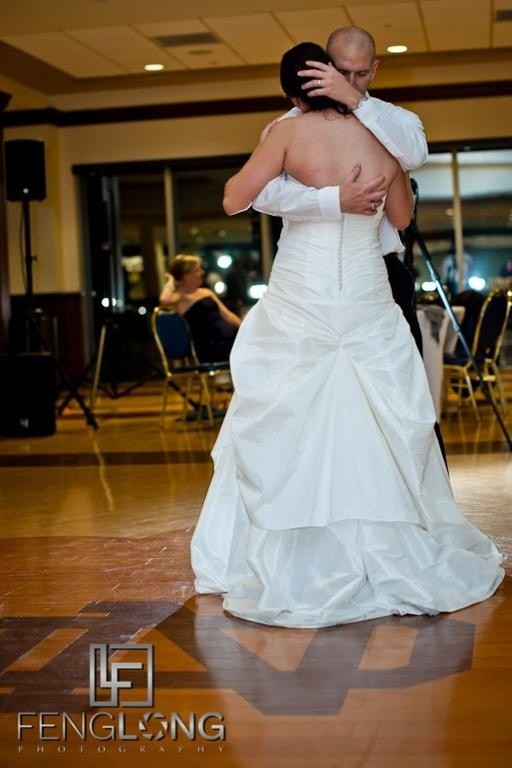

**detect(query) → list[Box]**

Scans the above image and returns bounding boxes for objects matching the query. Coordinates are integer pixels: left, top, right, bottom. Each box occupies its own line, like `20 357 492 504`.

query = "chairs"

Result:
150 304 233 430
416 288 512 421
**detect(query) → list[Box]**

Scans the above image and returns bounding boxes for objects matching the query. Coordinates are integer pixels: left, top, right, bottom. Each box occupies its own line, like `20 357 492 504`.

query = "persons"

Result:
440 239 473 297
187 40 506 628
159 254 244 363
251 26 451 478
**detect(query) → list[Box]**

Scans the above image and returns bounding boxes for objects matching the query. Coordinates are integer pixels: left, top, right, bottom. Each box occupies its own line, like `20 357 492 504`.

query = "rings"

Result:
318 79 321 87
371 202 376 211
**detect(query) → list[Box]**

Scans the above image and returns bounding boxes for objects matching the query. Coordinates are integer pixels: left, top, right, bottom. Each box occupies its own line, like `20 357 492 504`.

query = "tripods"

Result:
55 321 199 417
10 202 100 431
398 217 512 453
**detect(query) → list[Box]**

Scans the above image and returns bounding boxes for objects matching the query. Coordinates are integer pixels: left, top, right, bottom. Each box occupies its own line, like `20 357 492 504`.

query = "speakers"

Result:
2 137 49 203
3 314 57 437
